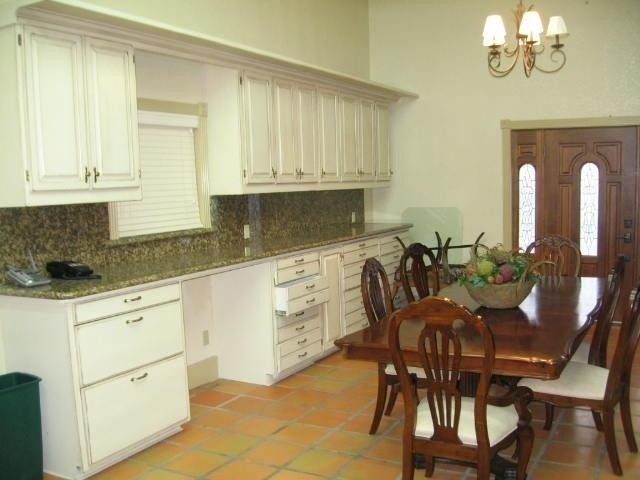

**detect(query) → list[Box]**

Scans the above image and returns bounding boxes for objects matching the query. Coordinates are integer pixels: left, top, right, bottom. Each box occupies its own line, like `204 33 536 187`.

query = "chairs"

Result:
360 256 456 434
523 234 583 279
511 287 639 480
543 253 625 431
399 243 440 302
385 294 533 480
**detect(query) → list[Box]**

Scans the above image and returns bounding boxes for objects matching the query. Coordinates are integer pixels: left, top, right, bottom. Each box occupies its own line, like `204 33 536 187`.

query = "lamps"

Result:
481 2 569 79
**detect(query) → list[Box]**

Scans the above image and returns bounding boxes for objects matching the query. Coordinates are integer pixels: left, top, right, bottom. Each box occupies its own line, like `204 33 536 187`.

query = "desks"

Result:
335 273 611 479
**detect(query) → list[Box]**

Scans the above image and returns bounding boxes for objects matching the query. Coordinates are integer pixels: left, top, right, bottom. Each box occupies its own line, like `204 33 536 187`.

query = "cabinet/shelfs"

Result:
0 19 144 212
360 92 392 190
340 235 380 338
211 251 330 386
381 226 412 312
320 86 361 192
0 278 191 479
274 75 320 193
204 63 277 197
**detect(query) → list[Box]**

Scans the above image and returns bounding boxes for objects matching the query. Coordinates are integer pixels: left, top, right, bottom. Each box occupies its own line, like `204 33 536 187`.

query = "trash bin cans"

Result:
0 370 42 480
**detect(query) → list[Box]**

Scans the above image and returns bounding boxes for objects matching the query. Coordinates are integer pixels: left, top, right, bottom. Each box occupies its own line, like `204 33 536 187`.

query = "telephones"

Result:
4 264 52 288
47 260 96 282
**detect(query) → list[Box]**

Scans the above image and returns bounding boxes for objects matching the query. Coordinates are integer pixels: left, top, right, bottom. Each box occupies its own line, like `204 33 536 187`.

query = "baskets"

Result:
464 265 539 308
